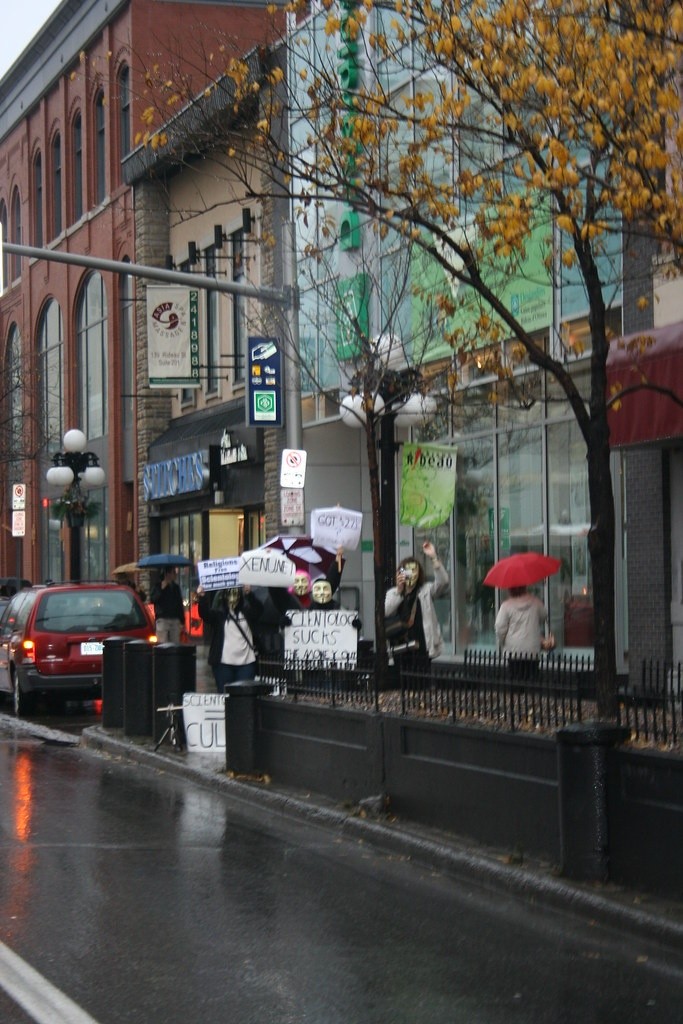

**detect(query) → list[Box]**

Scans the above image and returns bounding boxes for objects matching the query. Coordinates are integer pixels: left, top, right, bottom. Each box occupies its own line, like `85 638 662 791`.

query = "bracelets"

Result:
430 557 438 561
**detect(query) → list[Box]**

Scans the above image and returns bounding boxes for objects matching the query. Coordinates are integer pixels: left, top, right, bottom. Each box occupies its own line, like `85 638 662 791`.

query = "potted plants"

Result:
49 489 103 529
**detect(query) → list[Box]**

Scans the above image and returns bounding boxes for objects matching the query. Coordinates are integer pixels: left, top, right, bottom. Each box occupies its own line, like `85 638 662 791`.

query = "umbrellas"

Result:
483 552 559 588
136 554 195 576
112 562 149 585
260 536 346 576
0 577 32 587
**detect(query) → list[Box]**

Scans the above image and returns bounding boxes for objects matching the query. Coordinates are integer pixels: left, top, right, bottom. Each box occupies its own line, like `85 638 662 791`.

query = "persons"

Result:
384 541 449 691
150 567 185 643
495 586 548 681
0 585 16 596
278 578 363 683
117 572 147 602
197 583 264 690
288 545 346 608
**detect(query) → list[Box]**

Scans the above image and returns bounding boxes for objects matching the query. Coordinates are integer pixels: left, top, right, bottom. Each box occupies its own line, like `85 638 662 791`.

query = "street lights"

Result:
336 369 439 691
45 427 105 586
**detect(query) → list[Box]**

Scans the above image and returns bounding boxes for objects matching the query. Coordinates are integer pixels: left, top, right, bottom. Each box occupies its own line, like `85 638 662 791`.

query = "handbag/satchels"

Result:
382 586 420 655
538 617 558 651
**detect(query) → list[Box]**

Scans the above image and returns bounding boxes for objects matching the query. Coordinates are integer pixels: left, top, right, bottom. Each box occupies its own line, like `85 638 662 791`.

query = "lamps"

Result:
165 255 176 270
214 224 227 249
215 491 225 505
187 241 200 265
242 208 255 234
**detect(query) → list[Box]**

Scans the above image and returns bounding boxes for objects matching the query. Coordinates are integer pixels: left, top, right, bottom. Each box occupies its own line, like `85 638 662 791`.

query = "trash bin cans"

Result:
102 636 133 727
151 642 197 745
121 639 153 738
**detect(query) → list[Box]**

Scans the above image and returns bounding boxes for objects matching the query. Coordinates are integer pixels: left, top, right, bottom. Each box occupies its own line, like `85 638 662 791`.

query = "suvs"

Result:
0 578 156 717
